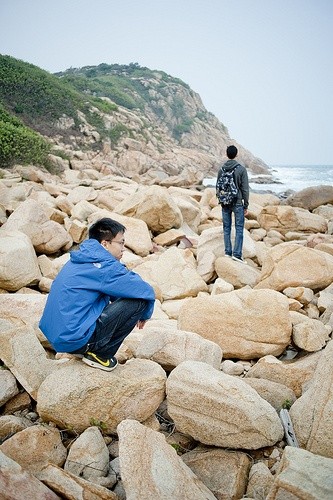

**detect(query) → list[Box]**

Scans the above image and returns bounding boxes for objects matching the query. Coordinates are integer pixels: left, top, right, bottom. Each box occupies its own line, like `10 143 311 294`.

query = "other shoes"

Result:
225 253 243 262
72 346 118 371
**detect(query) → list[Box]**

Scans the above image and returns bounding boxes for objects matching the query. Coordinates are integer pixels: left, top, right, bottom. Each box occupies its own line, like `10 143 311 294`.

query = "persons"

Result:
38 216 155 371
216 145 249 263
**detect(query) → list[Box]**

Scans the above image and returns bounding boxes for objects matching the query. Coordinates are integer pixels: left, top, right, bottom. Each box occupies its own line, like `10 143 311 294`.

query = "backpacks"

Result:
216 164 239 208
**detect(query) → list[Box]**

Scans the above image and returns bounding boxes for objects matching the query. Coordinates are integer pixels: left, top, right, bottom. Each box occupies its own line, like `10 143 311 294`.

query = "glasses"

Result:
105 239 124 245
